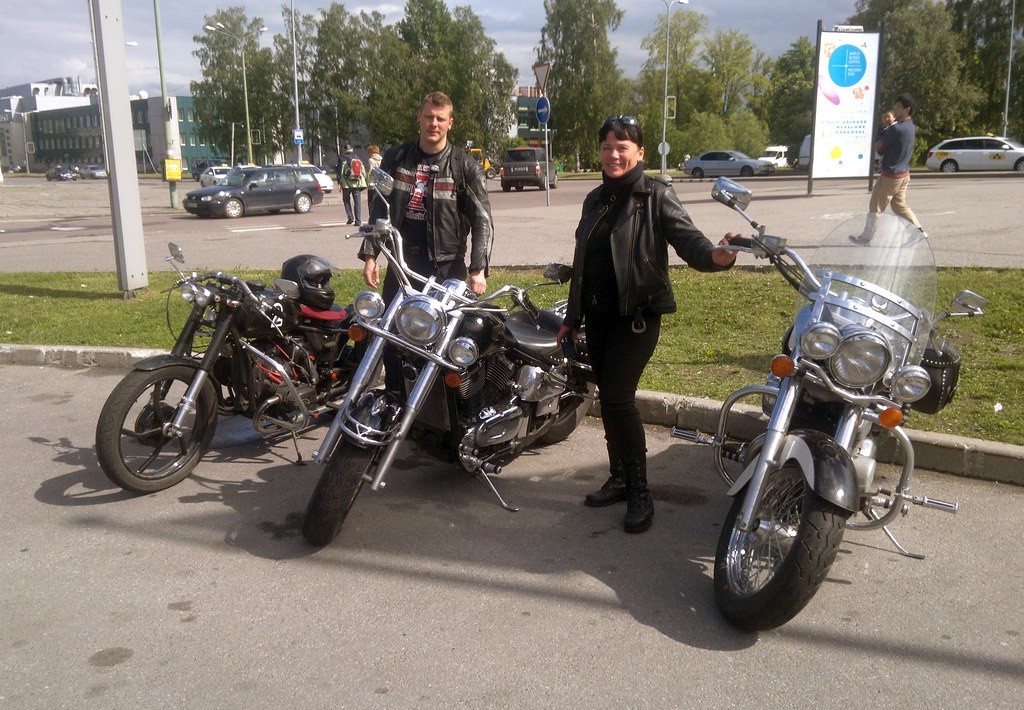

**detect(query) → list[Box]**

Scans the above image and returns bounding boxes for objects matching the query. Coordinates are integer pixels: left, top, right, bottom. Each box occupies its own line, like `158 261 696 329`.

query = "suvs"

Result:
182 166 324 220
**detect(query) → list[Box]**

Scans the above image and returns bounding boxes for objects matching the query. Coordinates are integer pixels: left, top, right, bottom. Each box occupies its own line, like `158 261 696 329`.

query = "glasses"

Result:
604 114 641 132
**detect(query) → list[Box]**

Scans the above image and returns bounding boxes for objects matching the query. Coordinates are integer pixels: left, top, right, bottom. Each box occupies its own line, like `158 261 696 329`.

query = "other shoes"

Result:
849 235 871 248
354 223 360 226
902 228 928 247
347 218 353 224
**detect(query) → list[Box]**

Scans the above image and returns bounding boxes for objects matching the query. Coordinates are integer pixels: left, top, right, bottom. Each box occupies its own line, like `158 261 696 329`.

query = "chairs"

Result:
254 174 265 186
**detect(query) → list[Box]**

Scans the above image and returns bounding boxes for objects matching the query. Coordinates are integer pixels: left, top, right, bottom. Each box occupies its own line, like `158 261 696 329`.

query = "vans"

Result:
796 135 882 173
758 146 790 168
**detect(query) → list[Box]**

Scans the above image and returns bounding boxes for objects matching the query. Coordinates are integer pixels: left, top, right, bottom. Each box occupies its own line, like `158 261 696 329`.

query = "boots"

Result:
585 442 627 507
619 454 655 532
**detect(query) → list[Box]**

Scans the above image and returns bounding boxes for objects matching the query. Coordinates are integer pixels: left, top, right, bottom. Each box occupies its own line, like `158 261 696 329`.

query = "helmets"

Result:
281 254 336 310
910 347 960 415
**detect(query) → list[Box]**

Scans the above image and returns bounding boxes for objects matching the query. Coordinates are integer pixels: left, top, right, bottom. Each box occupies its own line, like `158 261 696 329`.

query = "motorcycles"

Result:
97 242 387 494
301 166 603 549
669 176 991 633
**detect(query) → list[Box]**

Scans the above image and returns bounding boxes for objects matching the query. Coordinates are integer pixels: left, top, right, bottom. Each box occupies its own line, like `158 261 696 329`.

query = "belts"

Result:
405 246 420 256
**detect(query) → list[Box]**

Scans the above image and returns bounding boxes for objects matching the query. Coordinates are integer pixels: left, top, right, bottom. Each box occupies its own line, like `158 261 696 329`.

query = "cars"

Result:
79 165 107 179
682 150 775 178
500 147 558 192
471 149 496 179
46 164 79 181
191 158 333 194
924 135 1024 172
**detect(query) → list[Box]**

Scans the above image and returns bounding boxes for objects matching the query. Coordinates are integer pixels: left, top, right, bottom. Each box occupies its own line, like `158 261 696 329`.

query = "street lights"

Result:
205 22 269 165
655 0 691 182
4 109 39 175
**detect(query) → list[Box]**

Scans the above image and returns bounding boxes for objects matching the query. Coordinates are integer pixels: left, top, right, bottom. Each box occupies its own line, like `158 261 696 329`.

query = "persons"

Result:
556 115 743 535
848 93 927 249
337 142 367 226
357 92 495 441
367 145 383 216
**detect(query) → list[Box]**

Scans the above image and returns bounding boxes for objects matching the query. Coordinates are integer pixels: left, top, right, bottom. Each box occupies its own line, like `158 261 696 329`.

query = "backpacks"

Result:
343 154 362 181
